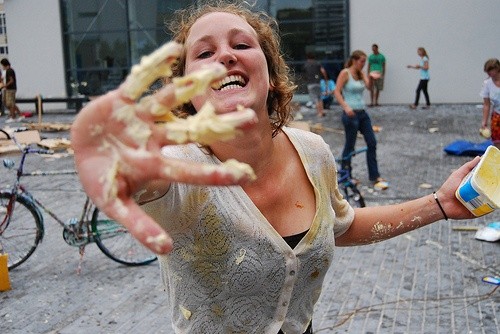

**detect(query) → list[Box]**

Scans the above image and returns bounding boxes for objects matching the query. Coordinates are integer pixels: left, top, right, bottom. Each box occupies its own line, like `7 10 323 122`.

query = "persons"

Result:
334 49 388 185
406 47 431 110
69 0 480 334
302 52 328 118
479 58 500 149
366 44 386 107
305 74 335 109
0 57 27 124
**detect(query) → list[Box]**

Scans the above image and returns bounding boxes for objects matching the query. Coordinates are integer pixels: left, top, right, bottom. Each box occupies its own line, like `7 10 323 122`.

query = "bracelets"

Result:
433 192 449 221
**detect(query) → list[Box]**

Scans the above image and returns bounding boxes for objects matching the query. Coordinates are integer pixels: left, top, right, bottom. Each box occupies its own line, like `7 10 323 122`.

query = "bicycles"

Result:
333 146 368 208
0 127 158 275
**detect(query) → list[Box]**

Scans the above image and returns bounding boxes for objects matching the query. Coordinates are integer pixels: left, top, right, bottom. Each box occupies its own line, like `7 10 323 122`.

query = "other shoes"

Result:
374 176 387 182
351 178 361 184
409 105 416 109
422 106 431 109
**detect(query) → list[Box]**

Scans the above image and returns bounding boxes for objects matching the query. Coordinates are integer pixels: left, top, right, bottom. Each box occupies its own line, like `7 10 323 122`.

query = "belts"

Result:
354 109 365 112
